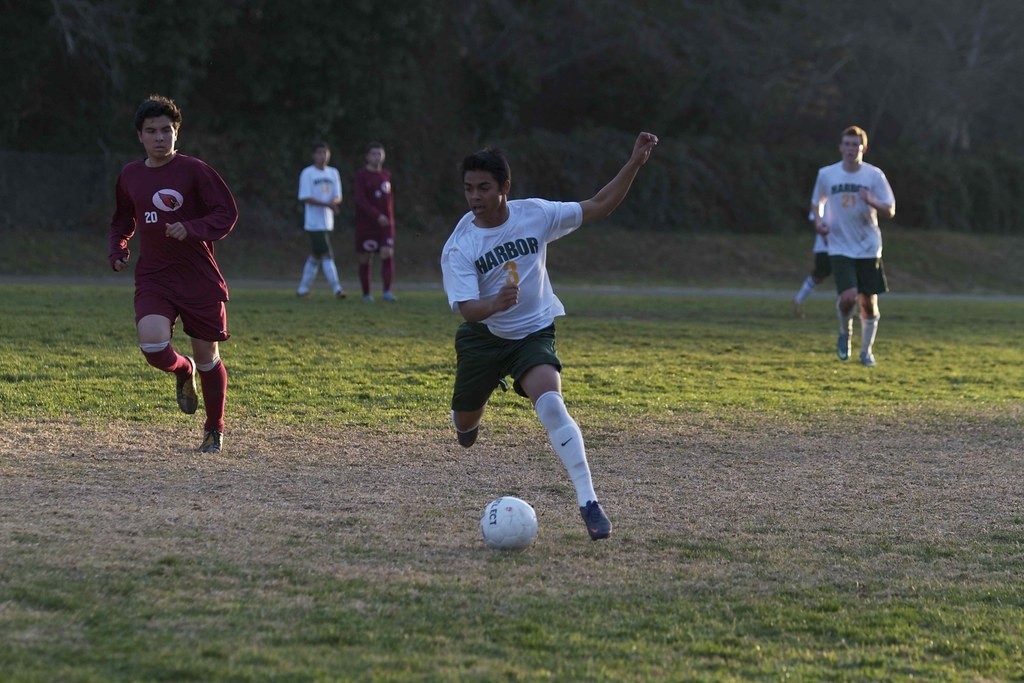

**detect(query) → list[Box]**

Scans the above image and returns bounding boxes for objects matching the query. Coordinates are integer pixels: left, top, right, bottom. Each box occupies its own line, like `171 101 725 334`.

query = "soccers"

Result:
480 495 539 552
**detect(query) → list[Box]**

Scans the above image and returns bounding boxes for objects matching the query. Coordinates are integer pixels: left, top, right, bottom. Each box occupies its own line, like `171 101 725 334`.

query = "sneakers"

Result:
199 428 223 454
860 352 876 366
579 500 612 539
837 332 853 360
457 426 479 448
176 355 198 414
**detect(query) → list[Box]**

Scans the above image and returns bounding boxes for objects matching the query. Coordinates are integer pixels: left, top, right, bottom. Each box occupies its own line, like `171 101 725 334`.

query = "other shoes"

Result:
336 291 347 299
296 292 310 299
363 293 375 301
792 297 805 319
383 290 396 302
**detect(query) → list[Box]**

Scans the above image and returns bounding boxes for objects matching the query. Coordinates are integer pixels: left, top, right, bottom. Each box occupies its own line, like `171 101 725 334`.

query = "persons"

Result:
296 143 346 299
353 142 396 301
109 96 238 453
792 126 895 368
440 132 659 540
